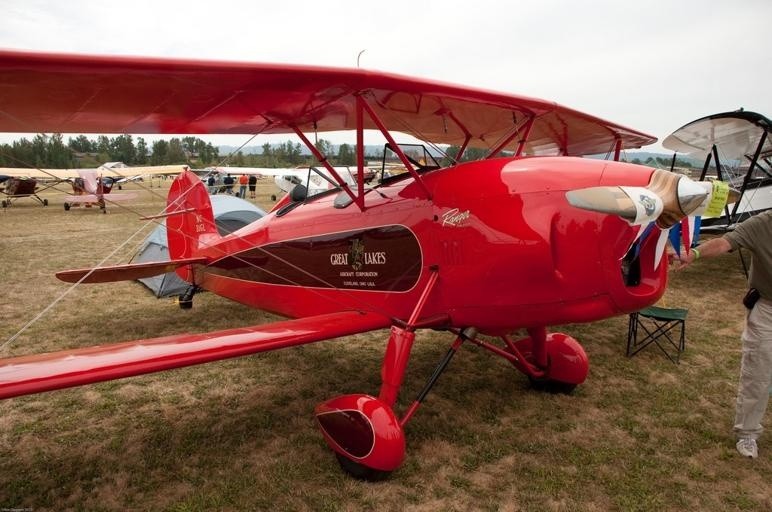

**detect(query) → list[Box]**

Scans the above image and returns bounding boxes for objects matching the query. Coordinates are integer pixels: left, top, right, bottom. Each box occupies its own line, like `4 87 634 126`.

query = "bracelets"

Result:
689 245 701 258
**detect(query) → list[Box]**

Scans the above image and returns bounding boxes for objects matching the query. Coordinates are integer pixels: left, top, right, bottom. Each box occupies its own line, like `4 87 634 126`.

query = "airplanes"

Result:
663 108 772 235
213 167 372 198
0 165 190 214
0 46 741 485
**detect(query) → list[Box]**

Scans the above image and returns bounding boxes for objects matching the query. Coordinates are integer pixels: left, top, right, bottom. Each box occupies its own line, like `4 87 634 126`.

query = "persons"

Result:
224 173 234 192
669 206 772 458
240 174 249 198
249 174 257 197
206 171 222 194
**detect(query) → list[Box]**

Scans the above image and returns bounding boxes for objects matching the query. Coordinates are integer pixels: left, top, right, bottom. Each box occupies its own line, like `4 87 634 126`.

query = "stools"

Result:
625 305 689 363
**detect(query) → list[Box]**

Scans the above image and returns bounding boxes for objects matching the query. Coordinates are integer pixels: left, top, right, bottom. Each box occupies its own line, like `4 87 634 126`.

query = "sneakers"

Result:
736 437 758 458
